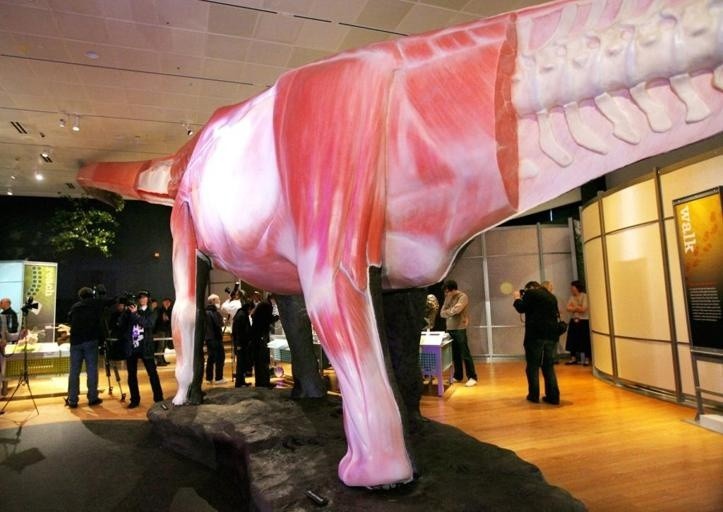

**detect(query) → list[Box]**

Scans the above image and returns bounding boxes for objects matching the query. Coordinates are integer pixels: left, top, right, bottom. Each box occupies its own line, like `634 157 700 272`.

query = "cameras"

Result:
21 297 38 317
520 289 525 295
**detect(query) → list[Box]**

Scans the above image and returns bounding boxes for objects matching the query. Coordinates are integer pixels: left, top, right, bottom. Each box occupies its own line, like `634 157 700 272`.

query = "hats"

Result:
139 290 150 297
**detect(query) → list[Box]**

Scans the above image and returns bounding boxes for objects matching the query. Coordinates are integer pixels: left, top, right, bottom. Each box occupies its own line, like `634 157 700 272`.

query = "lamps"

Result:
57 111 68 128
180 122 193 137
71 113 82 132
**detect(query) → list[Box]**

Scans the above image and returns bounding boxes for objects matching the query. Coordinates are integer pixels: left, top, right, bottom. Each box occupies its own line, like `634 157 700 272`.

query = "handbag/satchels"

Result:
558 320 567 334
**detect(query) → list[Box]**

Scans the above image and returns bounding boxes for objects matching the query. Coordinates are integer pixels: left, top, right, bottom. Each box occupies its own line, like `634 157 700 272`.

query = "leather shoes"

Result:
66 399 102 407
128 402 139 407
527 395 558 404
566 360 590 366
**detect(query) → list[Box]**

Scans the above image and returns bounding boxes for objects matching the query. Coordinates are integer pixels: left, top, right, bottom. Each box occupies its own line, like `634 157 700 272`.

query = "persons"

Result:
542 281 559 365
513 281 561 404
440 279 477 387
566 281 592 365
0 296 18 343
0 315 25 415
64 284 279 408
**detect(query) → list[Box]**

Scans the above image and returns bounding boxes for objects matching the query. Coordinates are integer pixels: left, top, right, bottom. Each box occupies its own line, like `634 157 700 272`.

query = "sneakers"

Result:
465 378 477 386
206 378 227 384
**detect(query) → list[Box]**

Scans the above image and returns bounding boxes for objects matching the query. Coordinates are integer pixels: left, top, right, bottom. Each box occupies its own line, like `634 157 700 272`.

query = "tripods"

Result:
1 320 40 416
